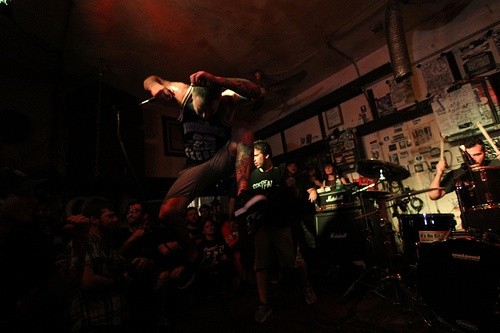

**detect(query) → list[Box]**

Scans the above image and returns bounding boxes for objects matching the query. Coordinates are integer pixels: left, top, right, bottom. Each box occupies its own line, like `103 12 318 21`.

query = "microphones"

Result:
461 145 476 165
139 90 172 106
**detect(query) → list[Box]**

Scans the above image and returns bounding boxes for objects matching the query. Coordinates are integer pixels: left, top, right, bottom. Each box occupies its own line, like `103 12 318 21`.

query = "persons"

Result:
0 166 161 333
246 140 318 323
429 137 500 201
184 201 228 269
142 69 266 288
315 163 347 188
282 156 319 272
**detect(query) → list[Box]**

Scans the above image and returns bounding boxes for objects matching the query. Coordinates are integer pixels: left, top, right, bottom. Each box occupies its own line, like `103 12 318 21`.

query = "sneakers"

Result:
305 286 316 304
234 189 268 218
178 247 205 290
254 306 273 323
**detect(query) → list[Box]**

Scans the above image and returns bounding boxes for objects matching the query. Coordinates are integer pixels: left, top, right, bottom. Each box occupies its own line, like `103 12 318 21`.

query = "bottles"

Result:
336 175 341 190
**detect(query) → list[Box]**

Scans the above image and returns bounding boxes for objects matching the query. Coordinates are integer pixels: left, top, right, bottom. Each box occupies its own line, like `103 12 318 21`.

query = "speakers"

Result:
314 209 398 271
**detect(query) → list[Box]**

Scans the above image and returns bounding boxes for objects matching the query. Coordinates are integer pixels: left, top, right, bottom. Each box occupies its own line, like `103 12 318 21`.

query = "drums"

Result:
401 213 456 262
414 232 500 333
454 166 500 231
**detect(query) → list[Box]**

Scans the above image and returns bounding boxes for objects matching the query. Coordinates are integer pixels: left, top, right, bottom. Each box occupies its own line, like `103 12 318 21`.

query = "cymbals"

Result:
356 190 398 197
356 159 411 182
385 189 436 201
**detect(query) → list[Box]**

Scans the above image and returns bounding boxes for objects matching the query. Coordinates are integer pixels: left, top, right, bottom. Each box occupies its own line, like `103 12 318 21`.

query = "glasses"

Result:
128 209 143 214
